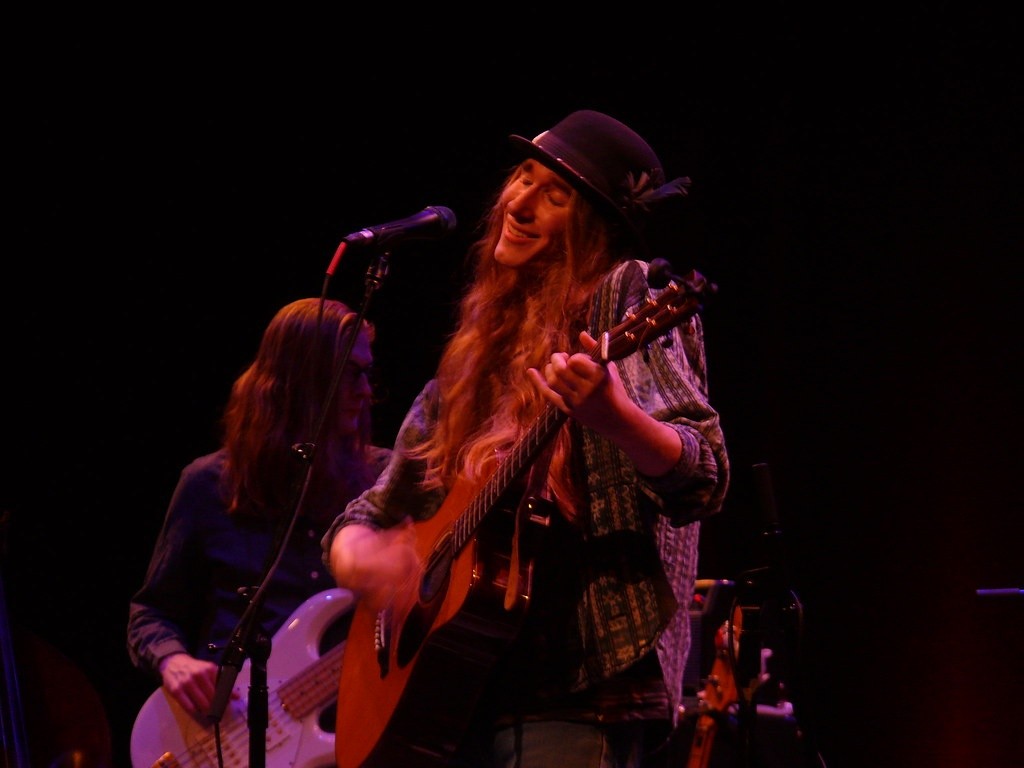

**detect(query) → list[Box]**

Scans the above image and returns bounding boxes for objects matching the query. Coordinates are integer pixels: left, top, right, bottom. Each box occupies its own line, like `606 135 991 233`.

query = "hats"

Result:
512 110 664 212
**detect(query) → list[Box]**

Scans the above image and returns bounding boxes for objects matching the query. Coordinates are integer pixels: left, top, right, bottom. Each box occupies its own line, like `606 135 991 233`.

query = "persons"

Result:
726 649 802 768
323 109 729 768
128 297 392 725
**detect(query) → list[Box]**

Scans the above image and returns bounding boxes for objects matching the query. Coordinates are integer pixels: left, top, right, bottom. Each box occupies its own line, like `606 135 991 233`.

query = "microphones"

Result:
344 206 457 249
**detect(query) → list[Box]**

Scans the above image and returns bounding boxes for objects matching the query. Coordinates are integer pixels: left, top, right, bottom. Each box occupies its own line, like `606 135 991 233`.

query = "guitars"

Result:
688 604 757 768
128 565 393 768
314 258 718 768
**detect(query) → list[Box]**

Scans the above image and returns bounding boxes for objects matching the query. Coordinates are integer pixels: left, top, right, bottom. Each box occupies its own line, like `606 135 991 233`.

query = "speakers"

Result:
681 578 737 696
